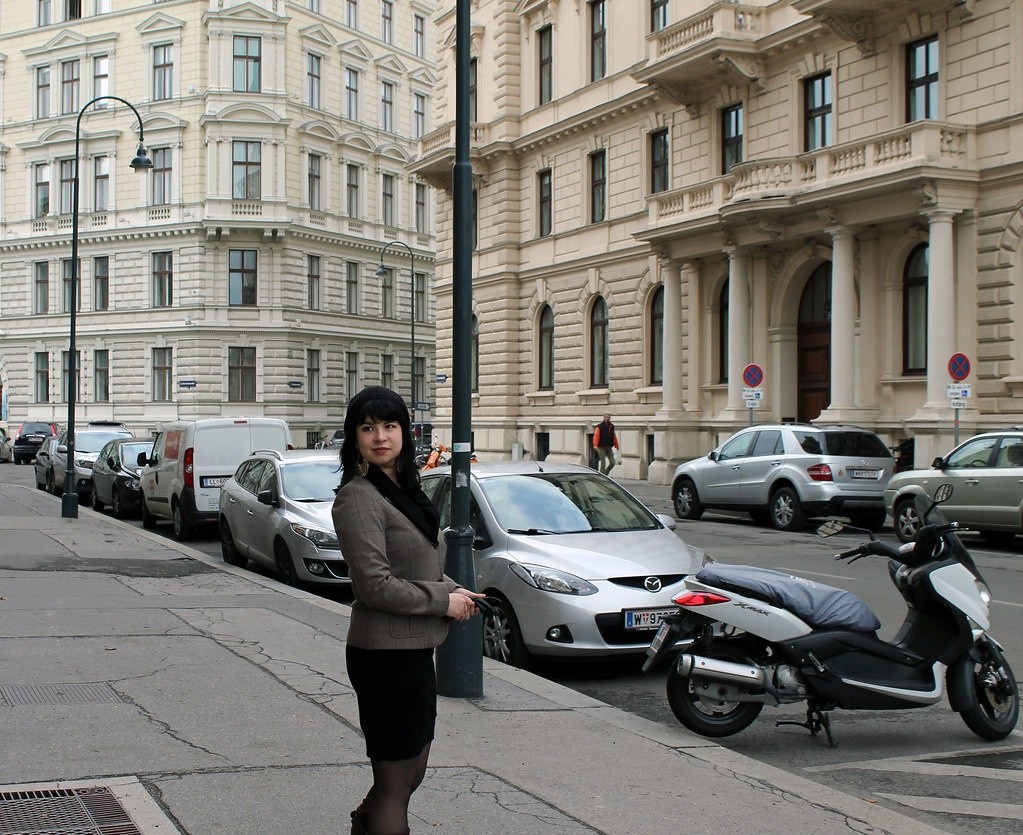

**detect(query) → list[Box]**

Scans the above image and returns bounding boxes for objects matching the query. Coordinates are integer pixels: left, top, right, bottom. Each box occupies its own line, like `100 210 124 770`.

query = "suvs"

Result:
13 420 64 465
49 421 136 507
671 422 896 534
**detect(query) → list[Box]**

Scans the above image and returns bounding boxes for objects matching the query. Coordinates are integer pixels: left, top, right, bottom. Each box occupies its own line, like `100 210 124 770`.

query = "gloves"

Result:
471 596 502 615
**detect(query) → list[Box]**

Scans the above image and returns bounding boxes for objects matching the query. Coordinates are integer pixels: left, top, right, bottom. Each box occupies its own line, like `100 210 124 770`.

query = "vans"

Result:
136 418 293 542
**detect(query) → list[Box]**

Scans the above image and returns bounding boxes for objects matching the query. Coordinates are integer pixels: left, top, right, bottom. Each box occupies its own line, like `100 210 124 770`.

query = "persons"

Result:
332 387 486 835
592 413 619 475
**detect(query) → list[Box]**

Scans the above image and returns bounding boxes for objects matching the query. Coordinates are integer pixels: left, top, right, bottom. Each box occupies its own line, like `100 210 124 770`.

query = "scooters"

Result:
640 484 1020 750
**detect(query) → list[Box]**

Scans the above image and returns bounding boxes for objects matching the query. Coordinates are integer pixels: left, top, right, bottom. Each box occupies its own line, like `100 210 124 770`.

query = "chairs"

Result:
1007 443 1023 467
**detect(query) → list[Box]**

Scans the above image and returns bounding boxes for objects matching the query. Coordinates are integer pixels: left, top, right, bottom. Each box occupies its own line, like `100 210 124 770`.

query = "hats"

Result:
347 385 410 422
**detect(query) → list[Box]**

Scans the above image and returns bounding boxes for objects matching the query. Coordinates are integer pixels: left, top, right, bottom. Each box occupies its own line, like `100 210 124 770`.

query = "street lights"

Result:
374 240 416 461
60 94 156 520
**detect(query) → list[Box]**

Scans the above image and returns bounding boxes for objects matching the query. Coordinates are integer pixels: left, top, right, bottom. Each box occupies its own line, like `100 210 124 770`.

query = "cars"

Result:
329 430 347 447
411 422 432 466
34 435 61 493
418 459 720 671
218 449 352 593
884 426 1023 545
90 435 158 520
0 427 12 463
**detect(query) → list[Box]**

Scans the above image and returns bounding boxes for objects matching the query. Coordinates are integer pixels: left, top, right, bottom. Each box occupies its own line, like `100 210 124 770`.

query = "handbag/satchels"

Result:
615 450 622 465
